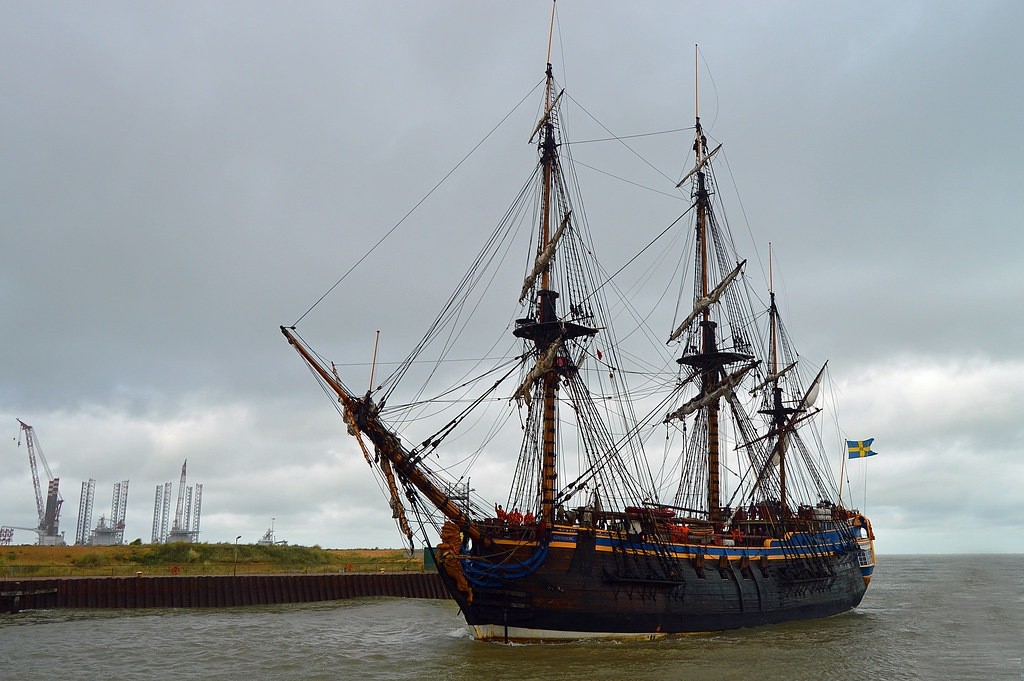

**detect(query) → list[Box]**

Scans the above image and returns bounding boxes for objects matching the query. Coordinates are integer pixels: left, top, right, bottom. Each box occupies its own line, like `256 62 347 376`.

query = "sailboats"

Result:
277 0 879 647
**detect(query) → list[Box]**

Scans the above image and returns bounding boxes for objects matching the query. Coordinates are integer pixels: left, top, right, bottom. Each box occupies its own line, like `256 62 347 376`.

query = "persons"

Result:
671 522 690 543
507 508 523 525
733 530 744 547
345 564 353 572
524 510 535 525
494 502 506 522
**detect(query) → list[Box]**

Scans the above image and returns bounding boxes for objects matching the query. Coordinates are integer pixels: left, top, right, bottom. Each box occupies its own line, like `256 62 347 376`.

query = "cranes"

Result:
169 459 194 543
13 417 65 549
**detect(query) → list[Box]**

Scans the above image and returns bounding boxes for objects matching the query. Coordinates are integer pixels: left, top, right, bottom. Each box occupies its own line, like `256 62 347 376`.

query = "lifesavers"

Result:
170 565 180 577
346 562 352 571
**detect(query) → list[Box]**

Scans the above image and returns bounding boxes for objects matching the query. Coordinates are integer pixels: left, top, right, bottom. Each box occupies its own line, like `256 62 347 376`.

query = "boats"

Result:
255 517 289 546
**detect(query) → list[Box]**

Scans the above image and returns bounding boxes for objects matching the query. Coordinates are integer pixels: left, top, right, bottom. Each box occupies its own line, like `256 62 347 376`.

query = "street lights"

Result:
233 534 242 576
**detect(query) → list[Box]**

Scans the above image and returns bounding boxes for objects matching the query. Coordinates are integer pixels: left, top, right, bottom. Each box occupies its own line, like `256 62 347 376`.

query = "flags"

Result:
847 438 878 459
